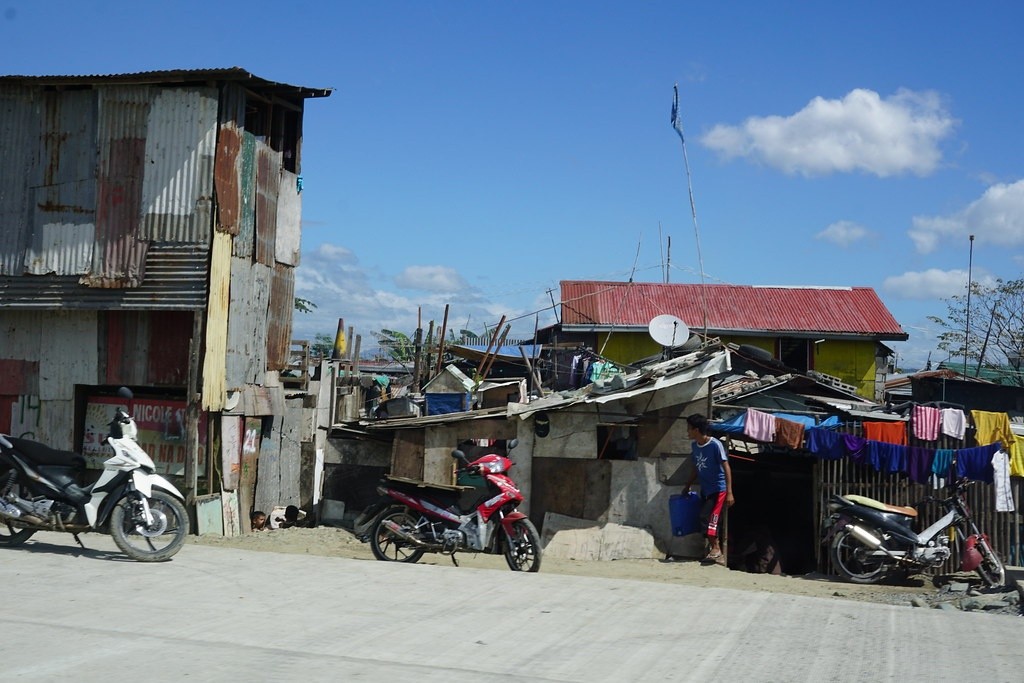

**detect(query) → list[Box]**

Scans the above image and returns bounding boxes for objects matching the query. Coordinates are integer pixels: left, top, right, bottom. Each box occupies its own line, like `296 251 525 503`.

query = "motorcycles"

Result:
0 386 190 563
820 448 1010 591
353 436 543 573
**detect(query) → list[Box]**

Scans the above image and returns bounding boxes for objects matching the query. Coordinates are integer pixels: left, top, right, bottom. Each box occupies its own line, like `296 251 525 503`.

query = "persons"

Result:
681 414 734 559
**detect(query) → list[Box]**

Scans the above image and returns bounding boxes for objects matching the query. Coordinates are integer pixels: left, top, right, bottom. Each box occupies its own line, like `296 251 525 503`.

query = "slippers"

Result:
706 550 722 559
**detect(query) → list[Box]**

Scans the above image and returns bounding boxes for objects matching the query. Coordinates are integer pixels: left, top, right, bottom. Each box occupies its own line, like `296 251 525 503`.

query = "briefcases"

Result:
669 489 702 536
